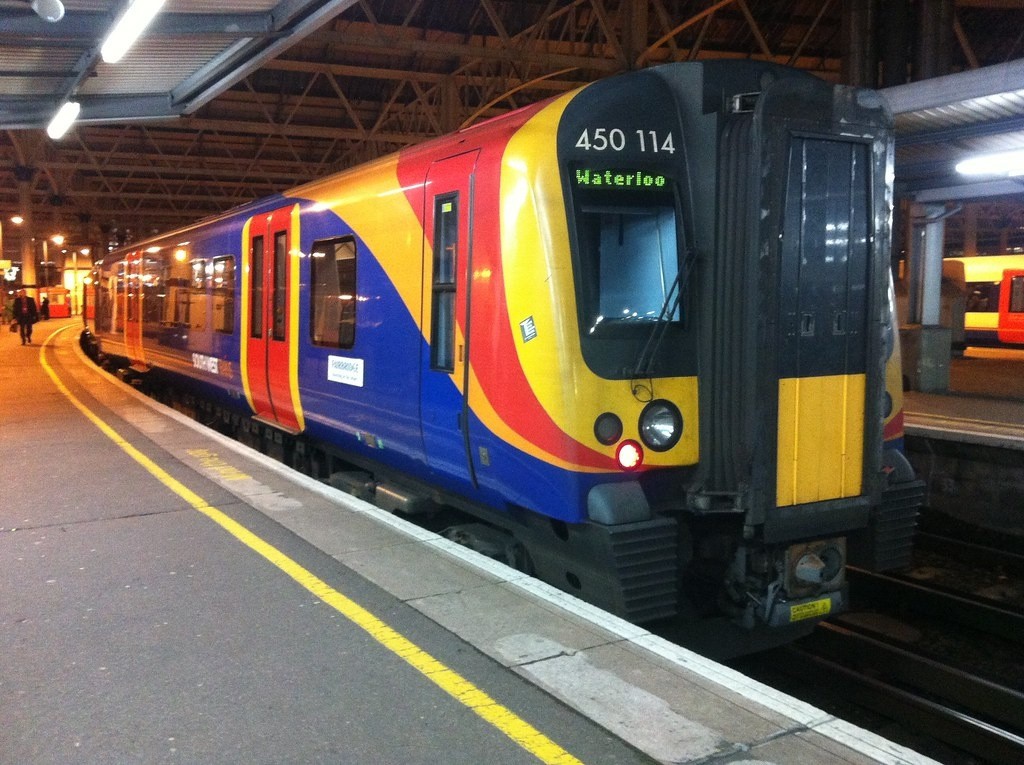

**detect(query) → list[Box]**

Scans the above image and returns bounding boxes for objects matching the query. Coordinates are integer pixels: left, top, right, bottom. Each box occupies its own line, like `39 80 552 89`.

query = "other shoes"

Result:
21 339 26 345
27 336 31 343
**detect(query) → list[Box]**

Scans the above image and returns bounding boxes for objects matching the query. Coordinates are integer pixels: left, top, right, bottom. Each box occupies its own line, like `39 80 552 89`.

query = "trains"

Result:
84 59 920 636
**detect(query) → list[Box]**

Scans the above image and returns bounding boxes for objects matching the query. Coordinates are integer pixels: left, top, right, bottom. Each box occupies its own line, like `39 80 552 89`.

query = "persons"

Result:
43 297 49 320
13 290 37 345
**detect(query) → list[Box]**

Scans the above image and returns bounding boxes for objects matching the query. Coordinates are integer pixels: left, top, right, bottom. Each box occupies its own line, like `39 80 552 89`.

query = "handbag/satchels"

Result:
10 319 18 332
32 312 40 324
